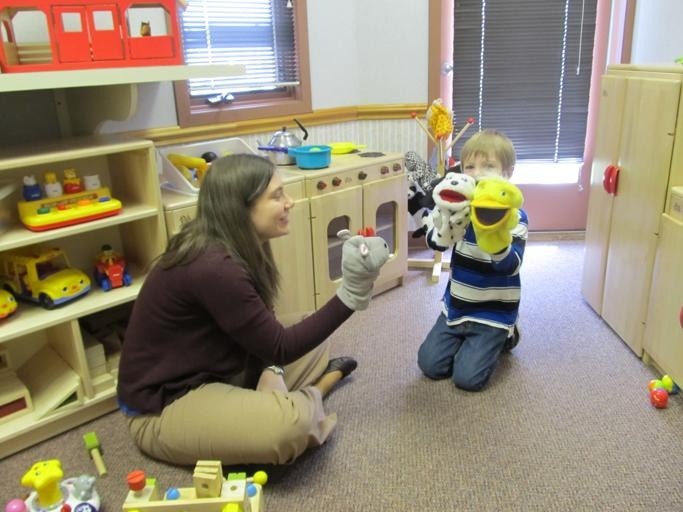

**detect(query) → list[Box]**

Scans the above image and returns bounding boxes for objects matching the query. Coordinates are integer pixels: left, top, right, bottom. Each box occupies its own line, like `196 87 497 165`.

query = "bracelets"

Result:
267 366 284 375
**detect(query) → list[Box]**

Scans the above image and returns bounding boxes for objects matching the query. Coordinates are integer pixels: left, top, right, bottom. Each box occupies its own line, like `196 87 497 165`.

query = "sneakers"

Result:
504 325 519 352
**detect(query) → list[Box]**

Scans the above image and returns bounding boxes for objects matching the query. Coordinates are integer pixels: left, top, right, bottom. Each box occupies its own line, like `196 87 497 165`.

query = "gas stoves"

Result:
288 138 405 197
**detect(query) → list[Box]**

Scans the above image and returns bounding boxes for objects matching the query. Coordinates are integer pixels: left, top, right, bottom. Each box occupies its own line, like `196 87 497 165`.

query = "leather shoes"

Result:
319 357 357 380
228 464 286 486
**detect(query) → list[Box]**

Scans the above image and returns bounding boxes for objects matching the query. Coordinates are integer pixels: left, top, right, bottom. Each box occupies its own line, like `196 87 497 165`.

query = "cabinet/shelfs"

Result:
580 64 683 391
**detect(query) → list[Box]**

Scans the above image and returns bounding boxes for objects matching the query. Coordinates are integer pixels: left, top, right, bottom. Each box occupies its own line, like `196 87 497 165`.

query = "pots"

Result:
256 145 333 171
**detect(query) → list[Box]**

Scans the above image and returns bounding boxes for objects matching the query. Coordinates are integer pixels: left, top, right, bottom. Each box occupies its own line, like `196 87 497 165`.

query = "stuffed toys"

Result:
423 171 477 248
412 210 450 252
470 179 524 254
405 150 442 192
409 186 432 218
335 229 390 312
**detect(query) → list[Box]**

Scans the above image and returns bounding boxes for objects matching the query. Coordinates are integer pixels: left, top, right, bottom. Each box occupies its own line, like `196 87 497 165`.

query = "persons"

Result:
417 129 528 393
116 153 358 484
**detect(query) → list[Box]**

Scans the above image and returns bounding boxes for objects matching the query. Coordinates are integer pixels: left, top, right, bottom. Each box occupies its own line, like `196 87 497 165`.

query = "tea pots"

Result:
254 117 309 166
